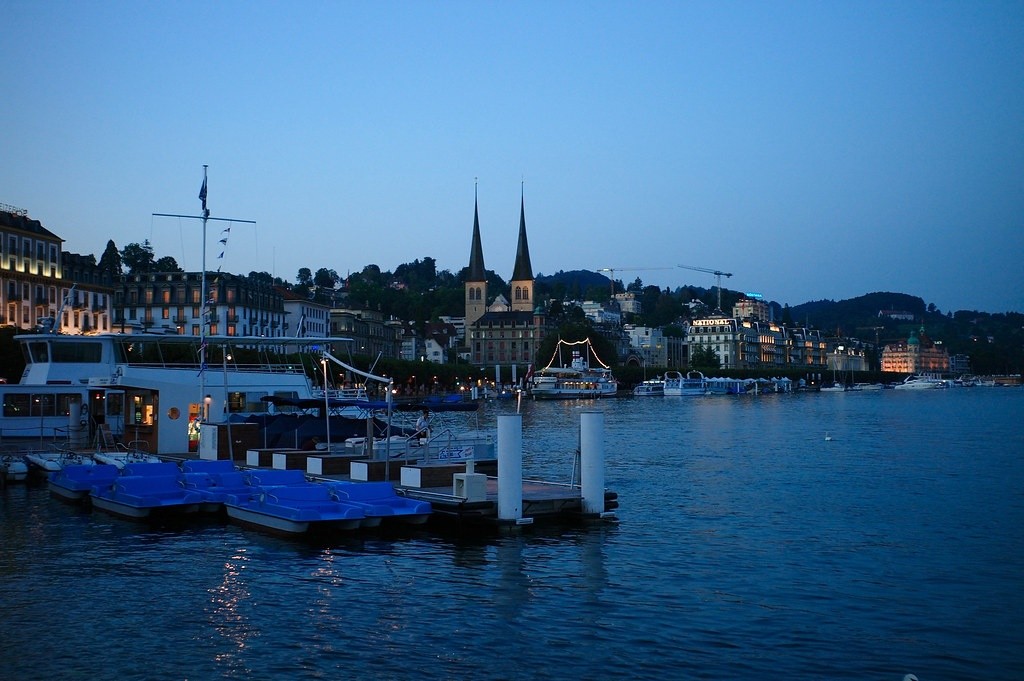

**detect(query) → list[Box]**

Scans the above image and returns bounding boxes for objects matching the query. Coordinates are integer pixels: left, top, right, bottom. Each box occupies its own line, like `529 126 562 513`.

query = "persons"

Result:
416 411 433 448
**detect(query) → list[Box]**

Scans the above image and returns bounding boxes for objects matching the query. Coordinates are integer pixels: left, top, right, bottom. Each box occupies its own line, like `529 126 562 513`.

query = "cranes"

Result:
677 265 733 309
596 267 672 292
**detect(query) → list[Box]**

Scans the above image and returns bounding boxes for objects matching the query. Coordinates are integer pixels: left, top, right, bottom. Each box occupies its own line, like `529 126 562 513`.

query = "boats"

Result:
747 385 817 395
333 409 498 472
0 452 29 482
858 374 999 390
0 317 435 452
487 391 513 400
310 389 398 410
46 459 434 540
92 451 162 471
634 383 663 396
422 395 464 403
530 333 619 399
663 371 707 395
24 451 96 478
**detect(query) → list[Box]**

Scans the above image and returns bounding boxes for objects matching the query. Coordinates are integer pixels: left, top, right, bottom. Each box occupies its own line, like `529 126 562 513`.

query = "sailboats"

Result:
820 350 862 392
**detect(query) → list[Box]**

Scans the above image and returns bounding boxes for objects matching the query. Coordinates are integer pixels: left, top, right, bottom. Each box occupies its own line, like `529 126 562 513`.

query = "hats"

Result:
314 437 320 443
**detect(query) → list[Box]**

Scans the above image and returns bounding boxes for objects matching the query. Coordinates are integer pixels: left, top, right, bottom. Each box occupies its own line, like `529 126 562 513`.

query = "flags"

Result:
199 176 207 210
